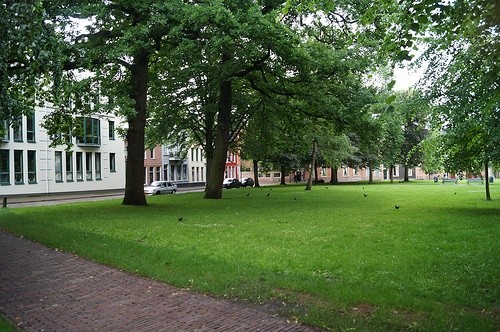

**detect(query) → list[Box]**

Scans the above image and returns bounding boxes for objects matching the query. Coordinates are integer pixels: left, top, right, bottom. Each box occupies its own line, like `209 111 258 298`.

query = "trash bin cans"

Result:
433 177 438 182
490 176 494 182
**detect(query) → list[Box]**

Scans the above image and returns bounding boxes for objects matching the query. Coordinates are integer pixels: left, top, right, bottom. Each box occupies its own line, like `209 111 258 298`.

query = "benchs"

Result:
466 177 485 185
441 177 458 184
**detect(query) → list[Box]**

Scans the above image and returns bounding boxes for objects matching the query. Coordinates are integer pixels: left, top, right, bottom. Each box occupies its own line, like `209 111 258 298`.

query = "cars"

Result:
222 178 242 189
239 177 254 187
312 179 325 184
144 181 178 196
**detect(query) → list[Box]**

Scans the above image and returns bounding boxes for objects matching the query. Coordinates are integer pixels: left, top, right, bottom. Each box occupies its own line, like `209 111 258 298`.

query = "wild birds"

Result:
362 187 364 189
270 188 272 190
260 189 263 191
363 193 368 198
245 192 250 197
394 204 401 209
177 216 183 221
324 186 328 190
454 192 457 195
266 192 270 196
293 197 297 200
